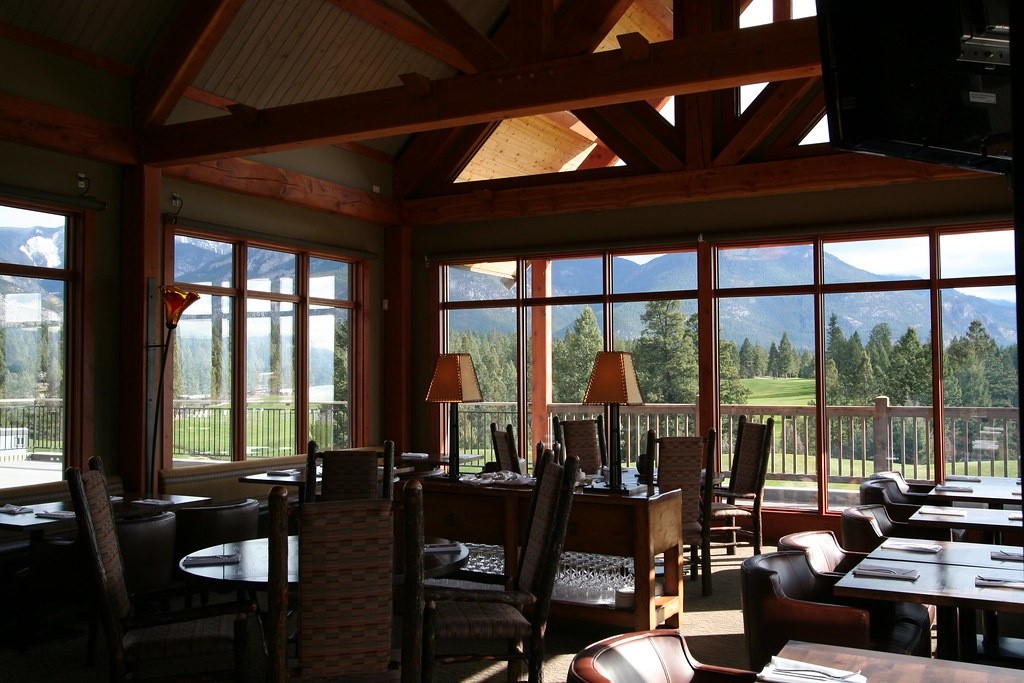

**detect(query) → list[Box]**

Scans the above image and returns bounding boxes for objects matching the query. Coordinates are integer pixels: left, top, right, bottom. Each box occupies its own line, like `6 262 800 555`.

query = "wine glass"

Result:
461 542 634 601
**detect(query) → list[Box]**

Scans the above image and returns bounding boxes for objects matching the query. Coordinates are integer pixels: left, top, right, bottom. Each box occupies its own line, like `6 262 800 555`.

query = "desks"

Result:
179 535 298 656
406 475 683 632
866 537 1024 571
909 504 1024 545
238 456 414 500
752 640 1024 683
928 485 1022 510
394 453 485 471
834 558 1024 662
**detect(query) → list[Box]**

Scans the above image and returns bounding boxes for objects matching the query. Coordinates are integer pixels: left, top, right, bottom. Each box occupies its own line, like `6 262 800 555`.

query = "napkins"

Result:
852 564 920 579
919 507 967 516
881 542 943 552
991 551 1024 560
935 484 973 492
975 574 1024 589
945 476 981 482
1008 513 1023 520
756 656 868 683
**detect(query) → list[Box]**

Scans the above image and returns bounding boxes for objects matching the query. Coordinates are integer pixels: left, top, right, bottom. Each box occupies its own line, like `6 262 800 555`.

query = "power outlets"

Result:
78 171 86 189
172 191 180 206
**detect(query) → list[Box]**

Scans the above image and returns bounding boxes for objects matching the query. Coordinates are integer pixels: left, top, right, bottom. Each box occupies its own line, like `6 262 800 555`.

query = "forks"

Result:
191 550 239 560
858 568 915 575
773 668 861 681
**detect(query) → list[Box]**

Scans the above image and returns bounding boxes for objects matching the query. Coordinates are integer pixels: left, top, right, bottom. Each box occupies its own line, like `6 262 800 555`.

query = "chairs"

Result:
421 449 579 683
553 415 606 471
268 479 420 683
683 414 775 558
490 422 522 475
66 455 249 683
645 428 716 596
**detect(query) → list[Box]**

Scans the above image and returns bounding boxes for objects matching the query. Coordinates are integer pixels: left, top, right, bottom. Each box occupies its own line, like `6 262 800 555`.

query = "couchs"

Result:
777 530 935 631
842 503 952 553
566 629 759 683
860 478 951 521
740 550 931 671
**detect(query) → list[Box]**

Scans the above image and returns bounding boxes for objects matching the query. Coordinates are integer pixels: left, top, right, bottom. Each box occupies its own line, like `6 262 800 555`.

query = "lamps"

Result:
149 284 200 498
424 353 484 482
582 351 648 496
